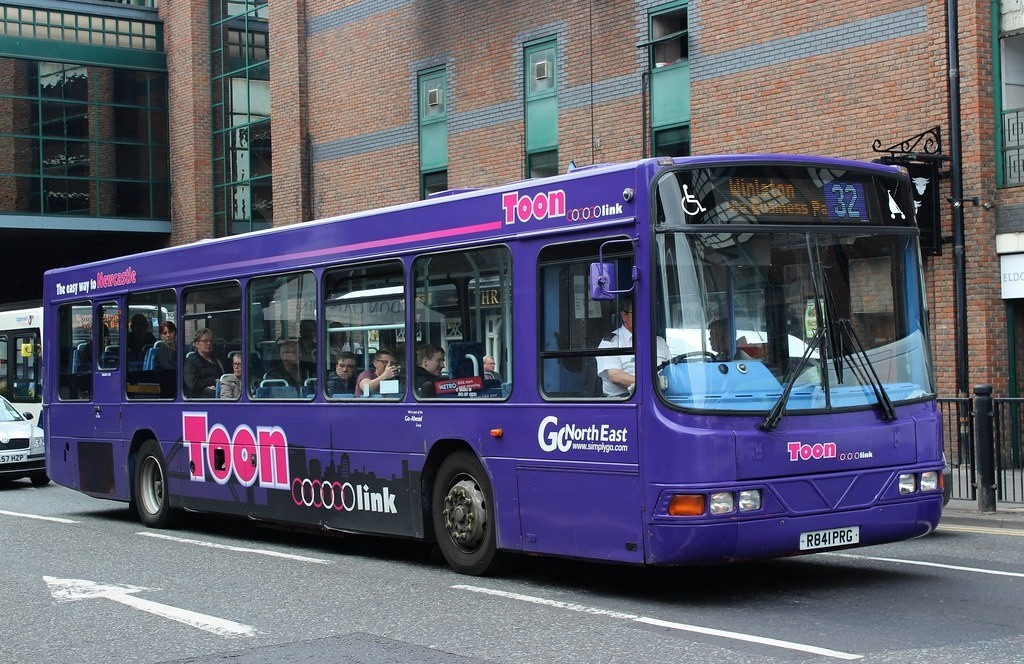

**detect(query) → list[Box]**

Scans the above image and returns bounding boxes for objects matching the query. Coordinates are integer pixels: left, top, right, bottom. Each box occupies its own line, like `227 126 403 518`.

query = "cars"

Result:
0 394 50 489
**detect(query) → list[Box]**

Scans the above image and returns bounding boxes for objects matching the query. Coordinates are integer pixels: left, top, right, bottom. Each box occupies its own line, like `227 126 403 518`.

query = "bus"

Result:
0 299 169 457
40 156 956 577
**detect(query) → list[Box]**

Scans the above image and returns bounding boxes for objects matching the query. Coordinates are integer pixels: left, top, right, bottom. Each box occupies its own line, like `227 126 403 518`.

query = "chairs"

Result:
72 339 501 399
581 336 604 398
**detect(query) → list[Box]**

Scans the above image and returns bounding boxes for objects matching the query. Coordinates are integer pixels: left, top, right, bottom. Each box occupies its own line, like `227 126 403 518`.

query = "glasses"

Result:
232 363 241 367
282 351 303 356
338 363 355 368
197 339 213 343
376 360 400 367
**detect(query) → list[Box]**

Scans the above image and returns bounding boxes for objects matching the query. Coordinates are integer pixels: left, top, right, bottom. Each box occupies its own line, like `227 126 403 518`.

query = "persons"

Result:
595 301 671 398
483 356 504 383
80 313 446 399
704 317 754 360
